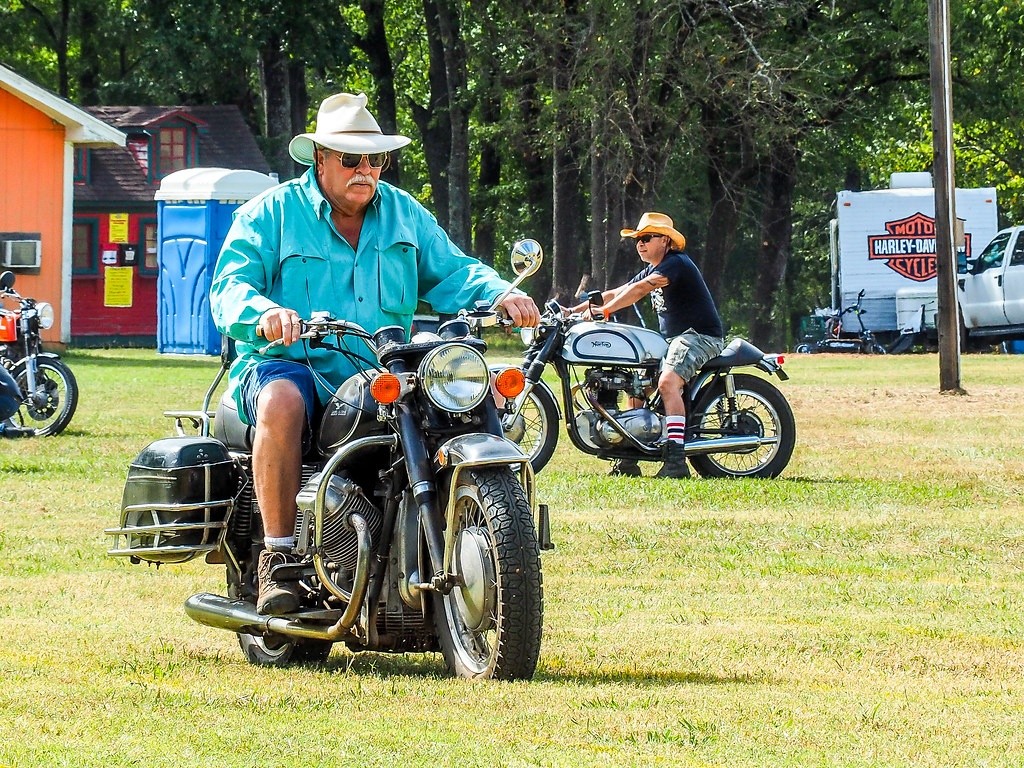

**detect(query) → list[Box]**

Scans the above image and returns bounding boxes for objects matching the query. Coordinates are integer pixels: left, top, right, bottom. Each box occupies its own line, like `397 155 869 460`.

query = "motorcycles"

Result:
488 286 797 480
0 270 79 438
104 241 556 684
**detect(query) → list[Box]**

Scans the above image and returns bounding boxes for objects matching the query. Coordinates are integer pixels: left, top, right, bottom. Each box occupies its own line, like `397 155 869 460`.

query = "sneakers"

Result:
256 545 299 615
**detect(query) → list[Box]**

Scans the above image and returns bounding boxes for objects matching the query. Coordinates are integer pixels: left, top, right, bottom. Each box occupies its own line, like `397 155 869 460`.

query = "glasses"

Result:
635 234 665 245
323 150 386 169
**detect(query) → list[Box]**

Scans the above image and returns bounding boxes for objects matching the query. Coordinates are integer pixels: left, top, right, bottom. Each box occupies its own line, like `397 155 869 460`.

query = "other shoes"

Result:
0 416 36 438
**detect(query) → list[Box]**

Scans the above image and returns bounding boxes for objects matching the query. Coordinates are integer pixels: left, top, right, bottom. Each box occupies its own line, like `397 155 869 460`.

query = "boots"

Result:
608 458 642 477
653 440 690 479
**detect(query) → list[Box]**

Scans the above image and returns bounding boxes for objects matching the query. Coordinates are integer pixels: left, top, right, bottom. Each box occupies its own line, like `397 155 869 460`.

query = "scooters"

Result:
795 289 886 355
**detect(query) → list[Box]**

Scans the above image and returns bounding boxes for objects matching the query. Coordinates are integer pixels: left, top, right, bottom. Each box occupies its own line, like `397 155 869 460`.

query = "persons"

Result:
208 92 541 615
550 212 724 479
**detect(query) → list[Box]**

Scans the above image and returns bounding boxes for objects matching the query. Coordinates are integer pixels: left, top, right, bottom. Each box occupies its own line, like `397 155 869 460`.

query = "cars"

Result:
956 225 1024 354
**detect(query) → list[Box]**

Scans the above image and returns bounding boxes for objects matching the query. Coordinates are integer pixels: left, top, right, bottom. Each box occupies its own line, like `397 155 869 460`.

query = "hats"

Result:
288 92 411 166
621 212 686 252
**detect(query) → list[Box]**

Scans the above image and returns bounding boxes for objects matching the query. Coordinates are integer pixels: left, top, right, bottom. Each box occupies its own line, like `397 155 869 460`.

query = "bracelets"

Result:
600 306 610 318
569 308 574 315
